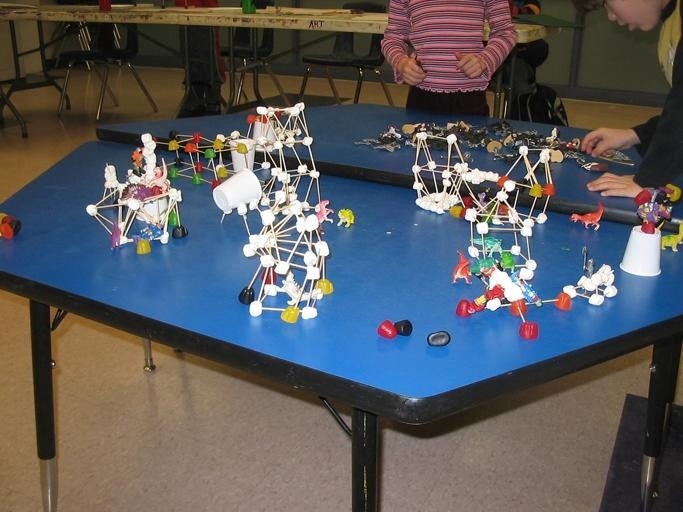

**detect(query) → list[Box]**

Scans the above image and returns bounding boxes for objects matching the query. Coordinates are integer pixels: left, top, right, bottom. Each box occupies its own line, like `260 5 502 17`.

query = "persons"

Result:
379 0 519 117
505 0 550 93
573 0 683 198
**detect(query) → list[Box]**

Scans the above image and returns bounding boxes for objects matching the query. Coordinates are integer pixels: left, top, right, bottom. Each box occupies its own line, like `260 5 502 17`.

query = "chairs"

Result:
297 4 398 107
47 3 158 119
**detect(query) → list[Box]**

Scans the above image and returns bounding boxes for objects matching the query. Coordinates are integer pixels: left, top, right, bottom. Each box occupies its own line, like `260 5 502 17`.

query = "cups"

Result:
618 224 663 279
212 168 263 214
252 120 275 153
229 138 257 173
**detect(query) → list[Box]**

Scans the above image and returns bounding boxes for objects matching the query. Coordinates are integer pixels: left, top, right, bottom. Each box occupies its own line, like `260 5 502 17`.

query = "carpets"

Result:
598 390 683 512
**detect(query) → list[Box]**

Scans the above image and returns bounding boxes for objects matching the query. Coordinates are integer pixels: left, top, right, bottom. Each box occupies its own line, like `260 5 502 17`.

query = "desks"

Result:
45 4 545 120
0 2 562 139
1 100 683 511
